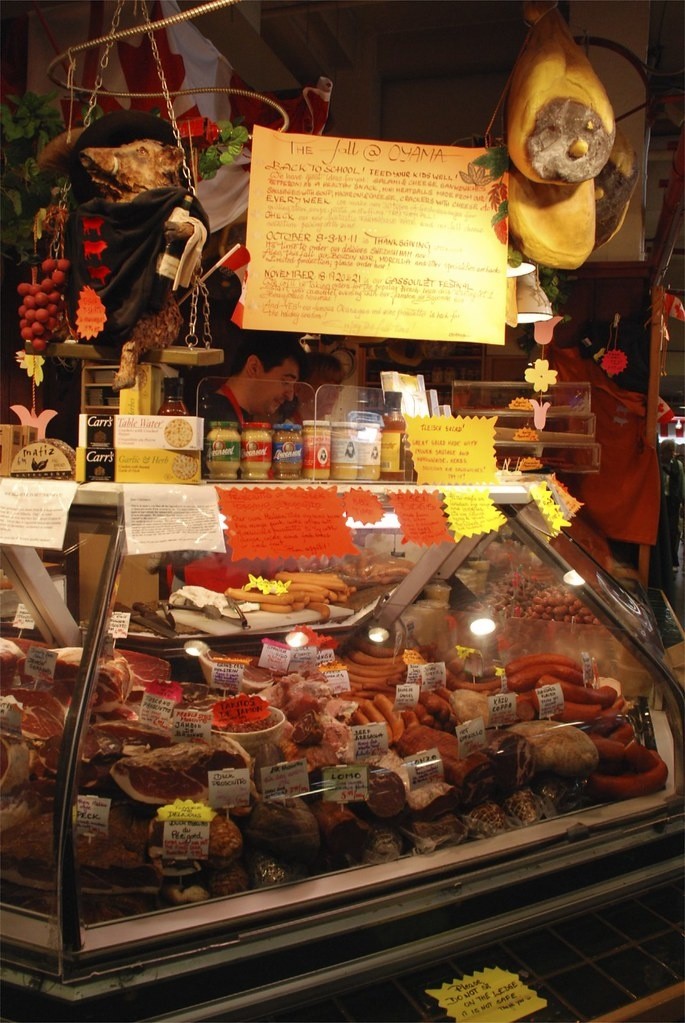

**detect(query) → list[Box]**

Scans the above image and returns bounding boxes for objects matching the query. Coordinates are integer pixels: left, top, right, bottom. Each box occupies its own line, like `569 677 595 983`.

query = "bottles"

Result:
380 391 407 480
157 383 189 415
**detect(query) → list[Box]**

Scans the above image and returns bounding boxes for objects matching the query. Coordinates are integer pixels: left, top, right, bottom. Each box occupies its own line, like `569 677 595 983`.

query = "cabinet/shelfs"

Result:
0 471 685 1022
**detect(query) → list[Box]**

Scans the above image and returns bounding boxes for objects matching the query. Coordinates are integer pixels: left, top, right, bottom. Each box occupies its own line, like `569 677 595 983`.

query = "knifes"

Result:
133 603 170 630
113 601 179 640
162 600 177 629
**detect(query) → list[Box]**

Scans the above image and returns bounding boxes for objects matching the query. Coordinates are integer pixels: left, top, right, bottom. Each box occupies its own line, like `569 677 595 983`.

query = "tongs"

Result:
204 573 248 627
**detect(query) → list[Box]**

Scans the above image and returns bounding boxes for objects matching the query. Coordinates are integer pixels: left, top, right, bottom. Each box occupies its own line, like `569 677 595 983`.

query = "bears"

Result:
63 138 208 392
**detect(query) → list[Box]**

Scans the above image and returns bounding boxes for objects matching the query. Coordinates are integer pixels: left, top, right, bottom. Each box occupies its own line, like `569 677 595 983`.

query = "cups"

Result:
426 585 452 602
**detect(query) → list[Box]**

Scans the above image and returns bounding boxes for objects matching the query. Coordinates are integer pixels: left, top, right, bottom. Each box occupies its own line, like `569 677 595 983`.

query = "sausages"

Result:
323 639 667 798
221 552 415 621
464 573 604 625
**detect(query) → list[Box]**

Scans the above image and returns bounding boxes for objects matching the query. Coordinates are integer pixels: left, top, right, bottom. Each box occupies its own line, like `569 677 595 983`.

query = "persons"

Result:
182 331 309 428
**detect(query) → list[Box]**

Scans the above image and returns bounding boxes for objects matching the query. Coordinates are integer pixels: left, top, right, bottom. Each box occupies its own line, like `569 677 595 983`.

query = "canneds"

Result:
204 418 384 481
